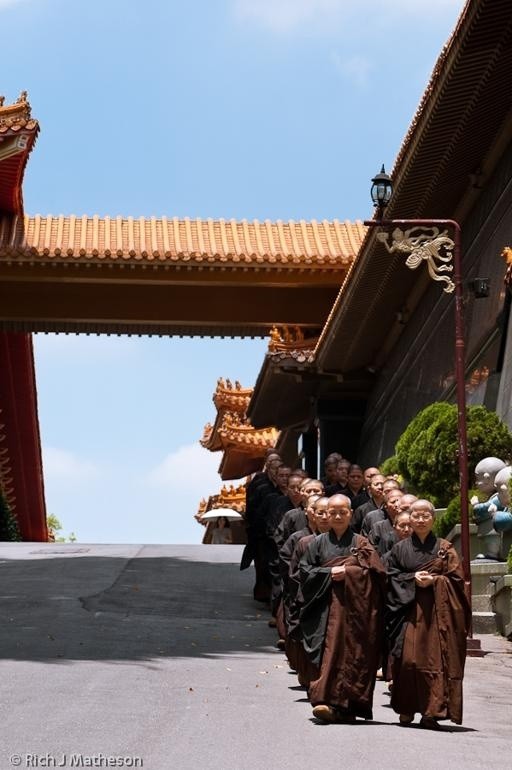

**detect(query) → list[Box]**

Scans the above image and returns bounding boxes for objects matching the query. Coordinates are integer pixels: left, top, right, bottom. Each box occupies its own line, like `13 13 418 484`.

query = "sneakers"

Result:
276 639 286 650
313 704 344 722
269 616 277 627
420 718 441 730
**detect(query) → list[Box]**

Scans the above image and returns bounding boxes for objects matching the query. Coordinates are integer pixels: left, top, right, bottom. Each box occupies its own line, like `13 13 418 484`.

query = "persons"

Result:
471 456 512 538
240 450 468 731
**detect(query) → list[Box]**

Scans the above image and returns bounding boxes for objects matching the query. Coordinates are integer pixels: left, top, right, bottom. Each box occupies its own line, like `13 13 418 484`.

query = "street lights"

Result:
364 165 489 657
